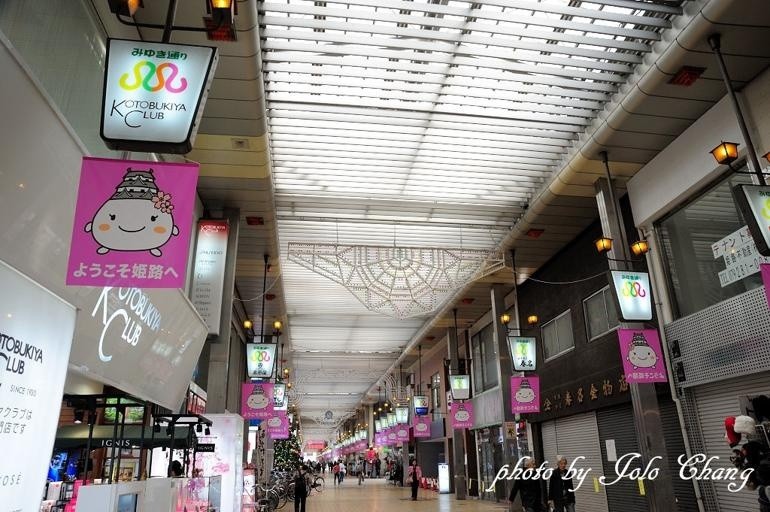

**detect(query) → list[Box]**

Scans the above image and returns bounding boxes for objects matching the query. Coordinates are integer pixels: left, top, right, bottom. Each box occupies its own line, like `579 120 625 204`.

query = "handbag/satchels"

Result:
406 471 414 485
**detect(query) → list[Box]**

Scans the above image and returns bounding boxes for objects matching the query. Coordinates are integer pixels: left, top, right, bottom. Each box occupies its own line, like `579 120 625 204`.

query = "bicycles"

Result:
252 468 326 512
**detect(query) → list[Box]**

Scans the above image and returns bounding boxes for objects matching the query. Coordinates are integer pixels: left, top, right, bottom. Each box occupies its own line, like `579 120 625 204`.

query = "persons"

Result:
408 459 422 501
275 460 284 474
307 453 402 485
293 465 307 512
508 455 575 512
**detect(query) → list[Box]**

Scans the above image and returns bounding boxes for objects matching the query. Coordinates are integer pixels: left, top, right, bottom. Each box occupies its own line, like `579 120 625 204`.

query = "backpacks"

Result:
295 470 307 490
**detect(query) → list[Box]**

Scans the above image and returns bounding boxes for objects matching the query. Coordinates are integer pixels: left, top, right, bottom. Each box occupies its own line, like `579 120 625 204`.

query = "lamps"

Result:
106 0 241 44
705 31 770 178
410 309 472 391
498 250 539 333
246 254 290 381
594 152 648 264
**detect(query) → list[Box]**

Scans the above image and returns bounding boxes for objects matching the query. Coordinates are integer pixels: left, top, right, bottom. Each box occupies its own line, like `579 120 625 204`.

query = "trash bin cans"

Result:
454 472 465 500
